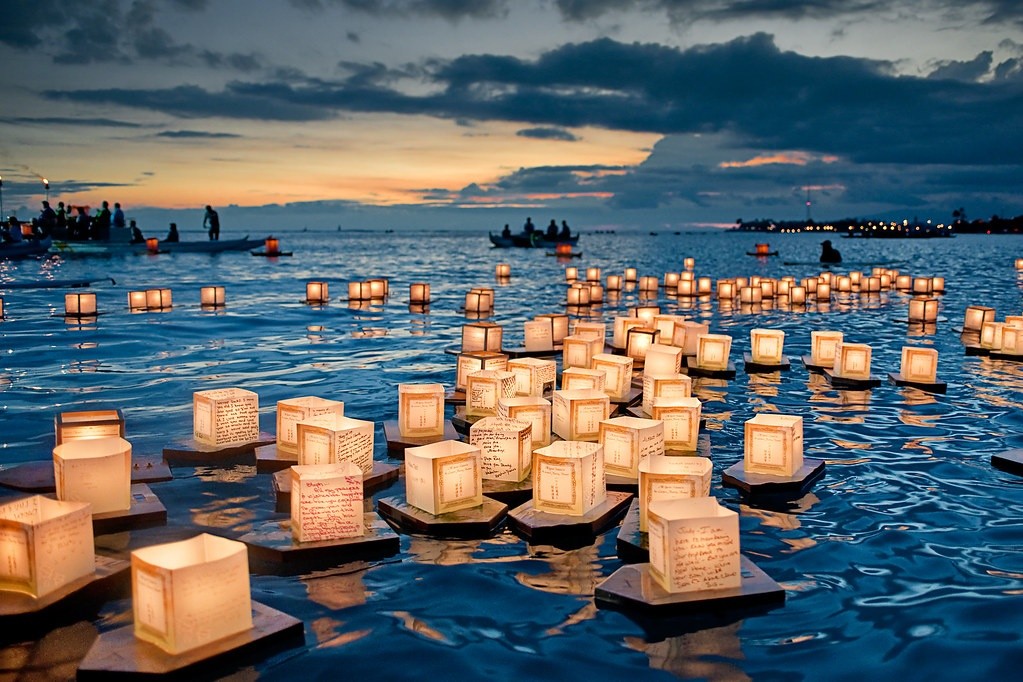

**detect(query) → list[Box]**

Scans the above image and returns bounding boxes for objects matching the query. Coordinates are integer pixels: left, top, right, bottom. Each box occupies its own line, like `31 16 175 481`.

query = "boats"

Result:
1 212 53 259
487 231 581 249
783 260 908 270
49 235 277 250
842 226 958 238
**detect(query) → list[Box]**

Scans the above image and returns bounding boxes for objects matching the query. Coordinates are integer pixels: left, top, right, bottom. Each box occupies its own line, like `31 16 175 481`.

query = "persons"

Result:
557 221 571 238
524 217 534 234
159 223 179 242
202 204 220 241
547 219 558 237
501 223 511 239
4 197 145 247
